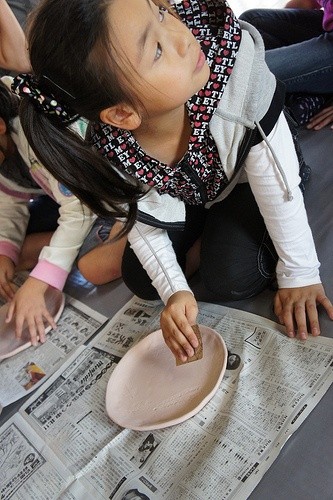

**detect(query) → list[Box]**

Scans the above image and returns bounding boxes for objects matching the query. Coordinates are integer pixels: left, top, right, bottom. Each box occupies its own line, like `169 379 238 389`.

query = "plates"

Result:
105 324 227 431
0 292 65 361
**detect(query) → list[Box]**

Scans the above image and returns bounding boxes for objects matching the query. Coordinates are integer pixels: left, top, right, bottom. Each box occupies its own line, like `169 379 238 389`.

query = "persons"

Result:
24 0 333 363
1 0 333 347
